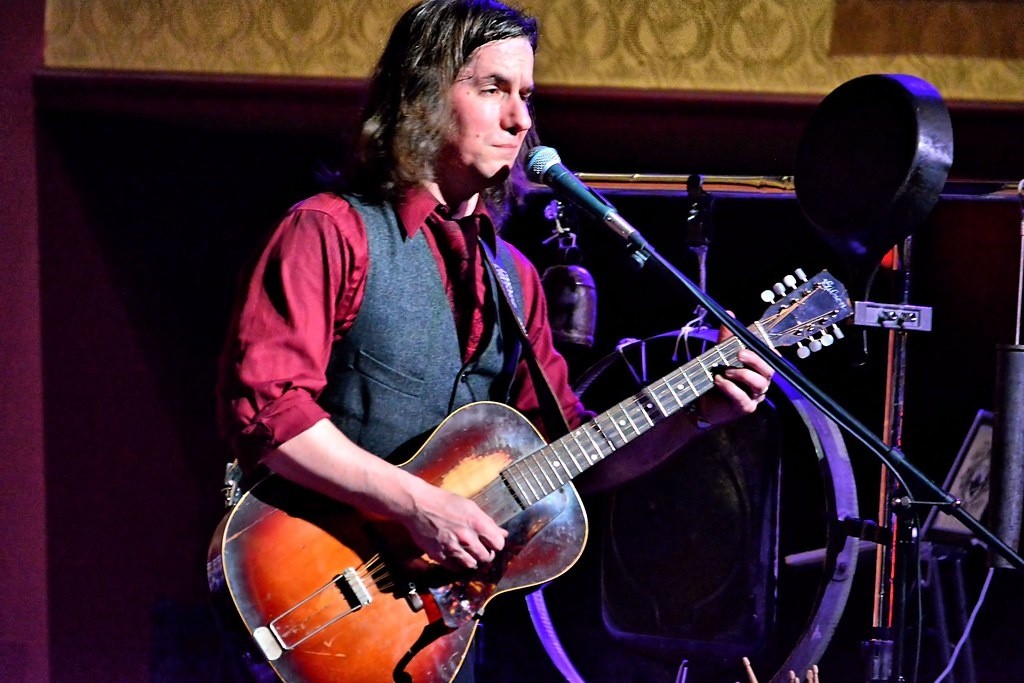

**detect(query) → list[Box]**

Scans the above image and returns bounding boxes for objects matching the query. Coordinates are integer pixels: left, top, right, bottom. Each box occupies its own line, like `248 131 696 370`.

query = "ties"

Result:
429 208 479 365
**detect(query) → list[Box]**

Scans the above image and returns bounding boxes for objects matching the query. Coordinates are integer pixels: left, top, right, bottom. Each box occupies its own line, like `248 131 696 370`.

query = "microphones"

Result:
522 145 647 250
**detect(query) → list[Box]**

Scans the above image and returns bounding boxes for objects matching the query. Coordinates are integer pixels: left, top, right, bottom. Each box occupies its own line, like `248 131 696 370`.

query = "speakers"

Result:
599 406 788 657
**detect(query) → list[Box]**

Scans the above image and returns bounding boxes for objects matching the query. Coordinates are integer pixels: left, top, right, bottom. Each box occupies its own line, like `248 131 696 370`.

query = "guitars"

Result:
205 267 856 683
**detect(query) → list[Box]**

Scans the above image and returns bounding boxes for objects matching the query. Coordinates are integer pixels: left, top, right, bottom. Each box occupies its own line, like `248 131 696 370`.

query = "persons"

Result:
218 0 780 682
